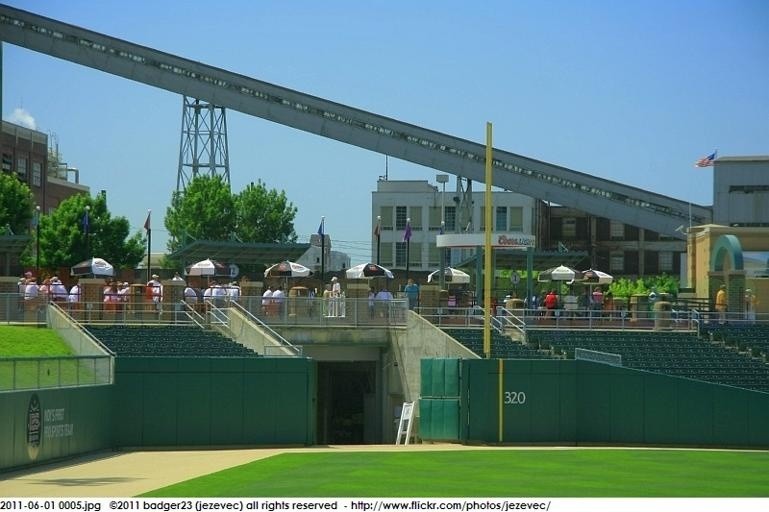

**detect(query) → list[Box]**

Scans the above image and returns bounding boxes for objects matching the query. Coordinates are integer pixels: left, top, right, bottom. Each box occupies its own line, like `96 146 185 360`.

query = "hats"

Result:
19 272 129 288
152 274 159 278
331 277 337 281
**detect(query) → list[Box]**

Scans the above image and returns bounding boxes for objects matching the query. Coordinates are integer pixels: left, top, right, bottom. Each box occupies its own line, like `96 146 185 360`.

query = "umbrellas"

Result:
427 266 471 290
263 259 312 287
576 267 614 296
71 256 117 280
537 264 581 301
184 257 228 285
345 261 395 292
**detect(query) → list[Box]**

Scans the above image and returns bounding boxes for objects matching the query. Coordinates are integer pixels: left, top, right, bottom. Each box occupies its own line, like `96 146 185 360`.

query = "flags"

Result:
143 214 150 233
402 222 412 242
694 152 717 166
81 211 88 234
317 221 324 237
373 222 381 240
32 210 39 226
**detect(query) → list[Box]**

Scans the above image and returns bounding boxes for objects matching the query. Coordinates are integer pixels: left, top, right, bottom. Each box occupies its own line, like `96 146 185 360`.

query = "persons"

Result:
714 283 731 325
743 288 758 324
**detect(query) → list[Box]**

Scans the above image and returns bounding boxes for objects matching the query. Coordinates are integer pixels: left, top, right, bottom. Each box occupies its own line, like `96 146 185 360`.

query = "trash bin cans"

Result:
439 290 449 314
289 287 311 320
128 284 146 316
506 299 524 328
630 293 672 331
